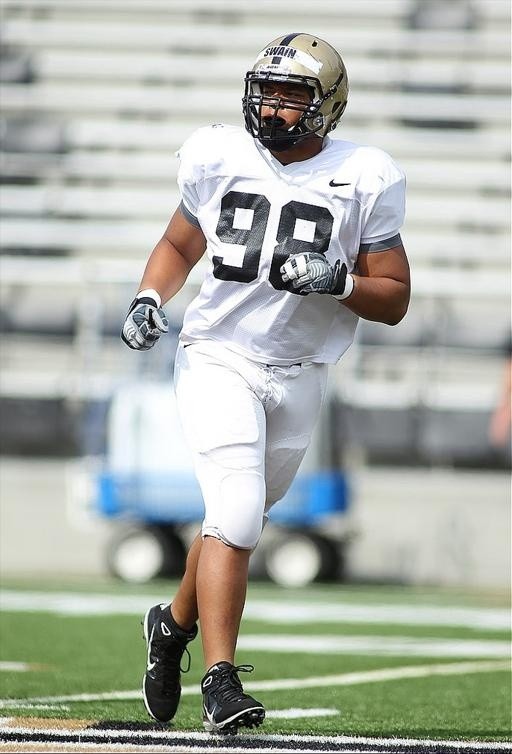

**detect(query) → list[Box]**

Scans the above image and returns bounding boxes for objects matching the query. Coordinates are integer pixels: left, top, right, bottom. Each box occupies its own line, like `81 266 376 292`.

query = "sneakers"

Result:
199 662 266 732
138 603 199 723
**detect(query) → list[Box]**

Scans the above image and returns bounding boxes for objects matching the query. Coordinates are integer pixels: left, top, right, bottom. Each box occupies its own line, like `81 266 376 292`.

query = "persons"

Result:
486 320 511 454
122 32 410 731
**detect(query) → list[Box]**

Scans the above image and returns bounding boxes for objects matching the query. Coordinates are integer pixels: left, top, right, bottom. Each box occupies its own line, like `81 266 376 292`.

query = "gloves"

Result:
276 251 348 298
119 288 171 350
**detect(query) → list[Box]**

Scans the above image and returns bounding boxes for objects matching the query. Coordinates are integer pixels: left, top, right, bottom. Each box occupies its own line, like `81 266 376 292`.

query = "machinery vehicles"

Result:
86 352 360 590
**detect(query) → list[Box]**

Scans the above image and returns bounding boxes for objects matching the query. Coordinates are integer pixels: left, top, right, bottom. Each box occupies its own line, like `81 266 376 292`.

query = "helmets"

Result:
241 31 348 151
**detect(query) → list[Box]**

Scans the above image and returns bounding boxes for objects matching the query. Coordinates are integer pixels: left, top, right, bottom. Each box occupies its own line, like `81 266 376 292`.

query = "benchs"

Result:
0 0 511 474
